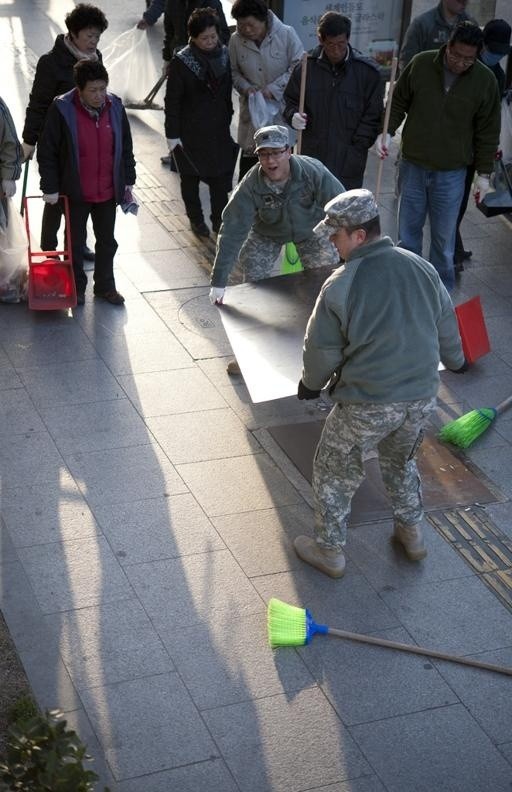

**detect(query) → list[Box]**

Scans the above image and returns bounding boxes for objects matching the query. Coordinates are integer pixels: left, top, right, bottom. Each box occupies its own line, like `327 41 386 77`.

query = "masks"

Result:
480 45 505 67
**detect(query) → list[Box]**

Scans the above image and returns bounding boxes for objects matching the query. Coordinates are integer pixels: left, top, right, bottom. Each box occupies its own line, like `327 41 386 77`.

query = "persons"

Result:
162 0 388 239
0 95 26 235
289 187 469 584
451 15 512 274
371 23 503 296
35 56 139 309
21 2 109 265
133 2 232 81
206 123 354 382
397 1 483 81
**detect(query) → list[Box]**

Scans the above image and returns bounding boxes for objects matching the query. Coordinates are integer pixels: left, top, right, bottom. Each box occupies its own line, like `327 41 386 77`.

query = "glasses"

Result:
258 148 290 160
326 45 346 52
448 55 475 67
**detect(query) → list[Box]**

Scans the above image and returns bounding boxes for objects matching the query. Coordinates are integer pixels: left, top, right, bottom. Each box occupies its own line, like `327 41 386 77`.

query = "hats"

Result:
252 124 290 154
482 19 512 56
312 188 379 241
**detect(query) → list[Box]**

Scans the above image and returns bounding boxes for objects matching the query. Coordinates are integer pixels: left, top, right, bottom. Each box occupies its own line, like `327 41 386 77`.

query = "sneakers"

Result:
293 535 345 579
161 156 171 165
190 223 209 236
226 359 241 374
95 289 125 305
78 294 86 305
392 521 427 562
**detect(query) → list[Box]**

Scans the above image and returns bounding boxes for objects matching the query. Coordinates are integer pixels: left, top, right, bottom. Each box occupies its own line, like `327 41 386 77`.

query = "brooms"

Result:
268 594 510 678
282 56 307 274
434 395 512 452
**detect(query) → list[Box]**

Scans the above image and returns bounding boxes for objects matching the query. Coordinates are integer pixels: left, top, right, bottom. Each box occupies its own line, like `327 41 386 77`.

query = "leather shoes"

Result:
464 249 473 258
84 246 95 261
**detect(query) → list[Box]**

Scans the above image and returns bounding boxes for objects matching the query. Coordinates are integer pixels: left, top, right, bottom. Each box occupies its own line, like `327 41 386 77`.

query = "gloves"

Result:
291 112 307 130
21 142 35 164
2 180 16 198
166 138 183 152
43 192 59 205
374 133 390 160
208 286 226 305
472 176 489 204
297 379 320 401
449 359 467 375
